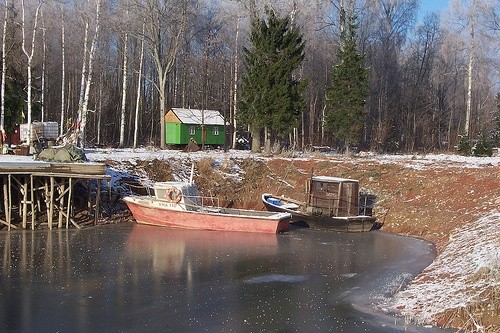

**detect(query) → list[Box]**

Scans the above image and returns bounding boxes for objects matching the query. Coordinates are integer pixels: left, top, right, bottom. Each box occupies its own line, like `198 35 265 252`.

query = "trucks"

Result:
9 125 49 149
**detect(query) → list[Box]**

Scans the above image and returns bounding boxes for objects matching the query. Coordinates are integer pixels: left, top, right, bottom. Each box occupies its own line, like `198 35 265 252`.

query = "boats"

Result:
262 166 378 232
123 183 292 234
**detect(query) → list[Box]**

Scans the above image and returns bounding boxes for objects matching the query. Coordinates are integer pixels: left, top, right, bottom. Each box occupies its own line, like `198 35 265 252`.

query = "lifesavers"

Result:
166 188 182 204
